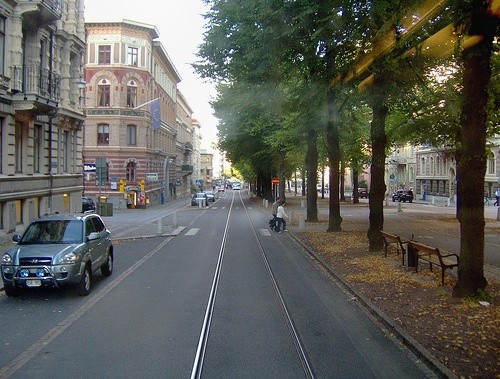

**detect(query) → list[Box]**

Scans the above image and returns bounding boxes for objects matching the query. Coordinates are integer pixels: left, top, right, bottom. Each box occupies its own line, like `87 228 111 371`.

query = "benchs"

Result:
381 231 408 267
411 240 460 286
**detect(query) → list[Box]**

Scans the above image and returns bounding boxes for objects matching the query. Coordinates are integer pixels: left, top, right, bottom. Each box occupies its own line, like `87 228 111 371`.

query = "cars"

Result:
191 193 209 206
358 188 367 199
204 191 215 202
217 187 225 192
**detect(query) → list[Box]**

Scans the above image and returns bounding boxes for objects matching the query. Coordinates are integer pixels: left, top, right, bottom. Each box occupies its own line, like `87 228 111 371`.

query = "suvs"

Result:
392 189 413 203
0 210 114 297
82 197 95 213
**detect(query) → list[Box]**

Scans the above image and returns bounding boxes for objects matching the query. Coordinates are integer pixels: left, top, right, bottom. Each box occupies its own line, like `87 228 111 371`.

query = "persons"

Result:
493 188 500 206
485 190 490 206
276 201 289 233
271 198 281 230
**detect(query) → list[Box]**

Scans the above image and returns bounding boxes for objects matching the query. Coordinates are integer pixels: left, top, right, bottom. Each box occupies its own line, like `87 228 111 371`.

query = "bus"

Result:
232 182 241 190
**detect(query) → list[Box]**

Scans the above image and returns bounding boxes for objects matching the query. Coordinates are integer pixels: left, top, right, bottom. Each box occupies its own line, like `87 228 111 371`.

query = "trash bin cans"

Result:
96 202 113 216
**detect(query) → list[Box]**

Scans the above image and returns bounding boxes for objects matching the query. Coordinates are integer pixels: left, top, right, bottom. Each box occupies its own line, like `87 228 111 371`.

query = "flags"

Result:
150 100 161 129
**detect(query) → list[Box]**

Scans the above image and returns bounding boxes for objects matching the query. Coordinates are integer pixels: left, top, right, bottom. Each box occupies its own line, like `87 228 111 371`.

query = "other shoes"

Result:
278 231 282 234
283 229 287 232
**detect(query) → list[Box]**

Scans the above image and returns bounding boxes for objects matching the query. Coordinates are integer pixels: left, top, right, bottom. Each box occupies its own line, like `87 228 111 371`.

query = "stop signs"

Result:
272 177 279 184
